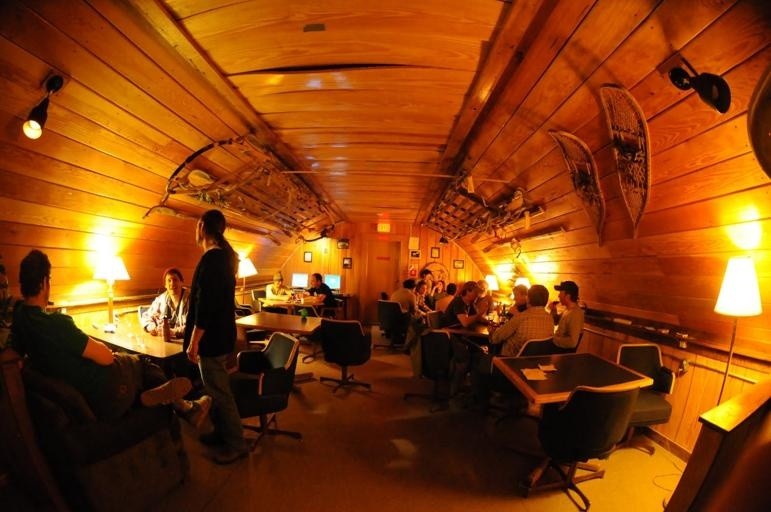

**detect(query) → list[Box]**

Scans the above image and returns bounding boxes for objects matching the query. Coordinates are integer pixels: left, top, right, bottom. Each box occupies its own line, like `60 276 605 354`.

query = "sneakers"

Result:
182 395 212 429
141 377 192 407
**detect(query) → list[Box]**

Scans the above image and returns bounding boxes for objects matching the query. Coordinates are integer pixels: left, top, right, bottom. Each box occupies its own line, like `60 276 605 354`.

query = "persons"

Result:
262 273 295 313
389 269 584 392
183 209 249 466
142 268 191 338
13 249 213 429
299 273 336 316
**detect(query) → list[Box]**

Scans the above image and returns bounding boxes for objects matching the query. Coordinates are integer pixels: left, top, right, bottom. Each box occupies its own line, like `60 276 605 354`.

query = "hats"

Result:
273 272 284 281
463 281 484 292
554 281 579 295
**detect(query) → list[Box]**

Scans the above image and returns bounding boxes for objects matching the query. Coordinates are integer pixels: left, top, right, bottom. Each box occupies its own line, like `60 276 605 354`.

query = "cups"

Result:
547 298 560 311
488 313 494 323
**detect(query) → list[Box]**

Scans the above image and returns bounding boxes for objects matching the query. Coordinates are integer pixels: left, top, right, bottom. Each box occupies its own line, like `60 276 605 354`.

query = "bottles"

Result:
499 303 506 324
162 314 171 342
301 307 308 323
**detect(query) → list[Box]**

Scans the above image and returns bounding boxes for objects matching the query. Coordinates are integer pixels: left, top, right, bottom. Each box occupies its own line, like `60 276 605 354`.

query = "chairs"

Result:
2 291 377 510
376 291 676 512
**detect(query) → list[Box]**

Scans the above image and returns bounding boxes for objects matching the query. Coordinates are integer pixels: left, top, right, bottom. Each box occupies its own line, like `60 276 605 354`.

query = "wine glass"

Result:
291 291 294 301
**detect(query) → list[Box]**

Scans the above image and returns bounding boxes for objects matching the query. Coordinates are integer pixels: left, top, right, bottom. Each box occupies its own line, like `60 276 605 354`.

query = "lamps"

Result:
712 256 763 407
484 275 499 296
236 258 258 290
21 68 64 140
93 256 131 324
668 49 731 114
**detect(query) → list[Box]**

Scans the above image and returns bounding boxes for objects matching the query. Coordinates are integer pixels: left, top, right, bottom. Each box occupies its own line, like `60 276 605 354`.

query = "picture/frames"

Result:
303 236 464 269
